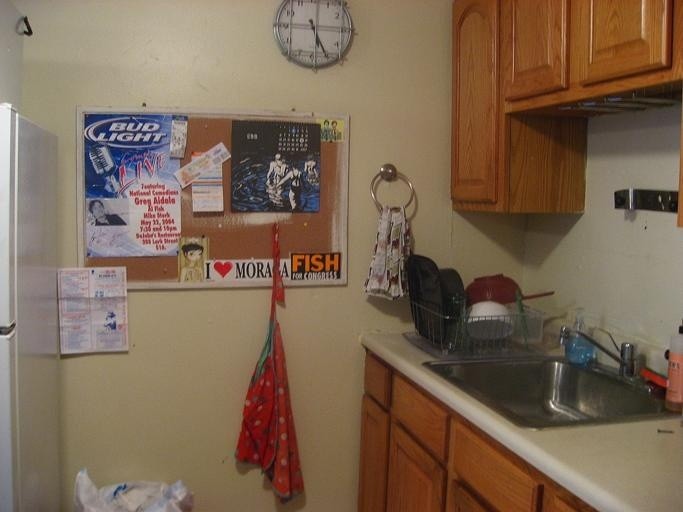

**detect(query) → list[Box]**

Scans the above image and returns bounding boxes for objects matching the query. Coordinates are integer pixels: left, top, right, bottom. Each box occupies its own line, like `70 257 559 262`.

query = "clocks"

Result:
273 0 353 73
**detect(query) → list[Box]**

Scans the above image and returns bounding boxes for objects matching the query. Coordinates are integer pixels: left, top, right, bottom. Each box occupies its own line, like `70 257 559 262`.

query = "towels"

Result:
364 203 412 301
235 222 304 505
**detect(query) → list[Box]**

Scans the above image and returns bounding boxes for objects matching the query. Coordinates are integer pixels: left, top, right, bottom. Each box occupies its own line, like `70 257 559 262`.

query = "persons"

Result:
285 189 308 213
302 153 318 178
265 152 286 181
331 120 341 141
87 199 126 226
178 237 203 282
319 120 331 140
276 159 307 189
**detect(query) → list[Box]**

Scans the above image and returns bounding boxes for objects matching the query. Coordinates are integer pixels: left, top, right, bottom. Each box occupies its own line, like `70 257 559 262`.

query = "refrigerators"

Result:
1 98 65 509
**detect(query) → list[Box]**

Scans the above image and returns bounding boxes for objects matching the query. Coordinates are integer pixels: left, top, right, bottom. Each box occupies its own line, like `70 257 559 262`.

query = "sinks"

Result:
422 354 683 431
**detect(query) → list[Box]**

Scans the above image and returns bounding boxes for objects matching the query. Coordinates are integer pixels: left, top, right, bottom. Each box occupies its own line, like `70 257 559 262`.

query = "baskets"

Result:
400 295 549 355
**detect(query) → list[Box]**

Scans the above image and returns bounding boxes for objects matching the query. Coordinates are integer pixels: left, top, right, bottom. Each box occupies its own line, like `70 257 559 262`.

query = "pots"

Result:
466 272 555 303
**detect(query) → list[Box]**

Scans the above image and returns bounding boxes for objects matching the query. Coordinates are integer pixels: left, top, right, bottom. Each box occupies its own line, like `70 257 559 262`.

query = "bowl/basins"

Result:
468 301 514 341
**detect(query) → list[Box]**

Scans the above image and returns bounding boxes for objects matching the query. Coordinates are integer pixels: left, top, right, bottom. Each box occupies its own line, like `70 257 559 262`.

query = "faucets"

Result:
559 324 635 377
